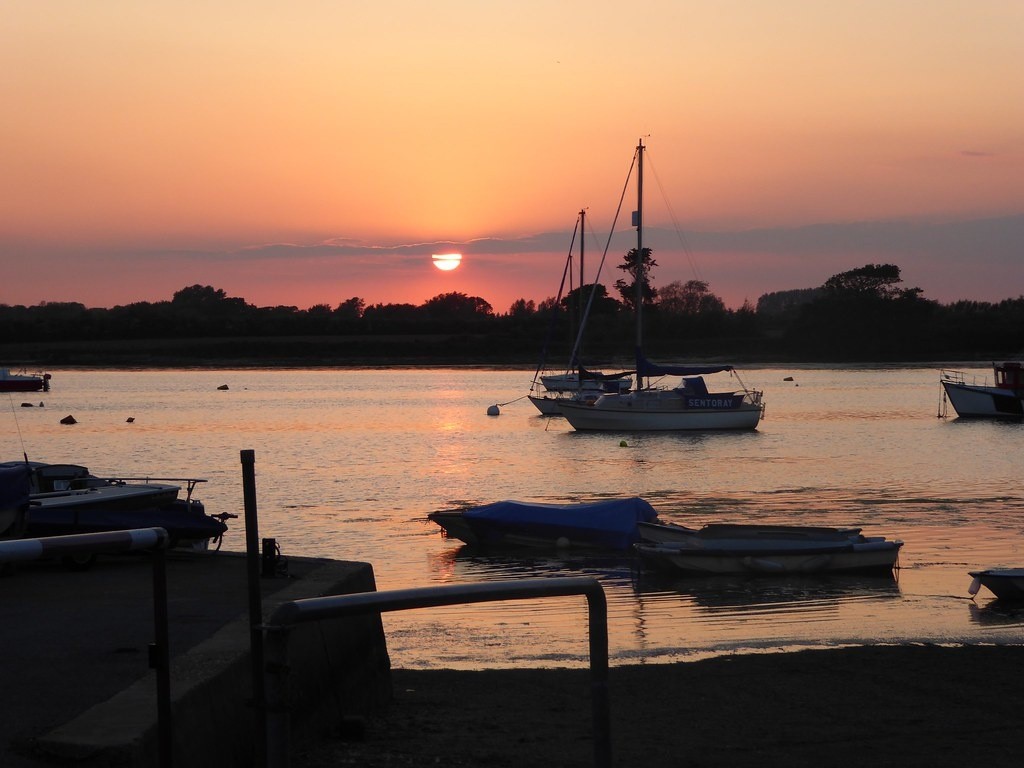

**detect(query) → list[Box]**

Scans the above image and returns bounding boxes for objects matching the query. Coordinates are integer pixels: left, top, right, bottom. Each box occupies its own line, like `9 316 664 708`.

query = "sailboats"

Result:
526 138 766 429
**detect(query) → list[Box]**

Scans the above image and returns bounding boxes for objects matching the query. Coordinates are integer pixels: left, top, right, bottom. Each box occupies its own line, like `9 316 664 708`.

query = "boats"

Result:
0 455 231 552
940 357 1024 422
630 535 906 580
636 519 864 542
425 496 666 559
966 567 1024 607
0 364 54 392
